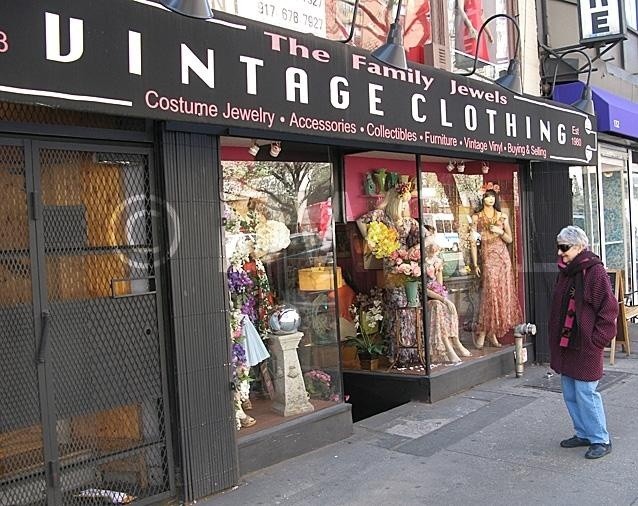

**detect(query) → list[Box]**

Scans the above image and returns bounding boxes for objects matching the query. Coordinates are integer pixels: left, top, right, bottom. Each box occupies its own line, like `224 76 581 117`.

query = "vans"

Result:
421 212 481 253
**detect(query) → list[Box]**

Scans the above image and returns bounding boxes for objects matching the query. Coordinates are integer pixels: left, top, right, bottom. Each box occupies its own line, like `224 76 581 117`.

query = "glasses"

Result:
557 245 576 253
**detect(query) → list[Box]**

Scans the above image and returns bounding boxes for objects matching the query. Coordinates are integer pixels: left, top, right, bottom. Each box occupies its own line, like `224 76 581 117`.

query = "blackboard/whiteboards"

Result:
603 269 621 352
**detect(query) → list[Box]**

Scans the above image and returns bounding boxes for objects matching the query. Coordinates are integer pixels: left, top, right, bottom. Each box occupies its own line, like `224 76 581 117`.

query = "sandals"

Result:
241 398 256 428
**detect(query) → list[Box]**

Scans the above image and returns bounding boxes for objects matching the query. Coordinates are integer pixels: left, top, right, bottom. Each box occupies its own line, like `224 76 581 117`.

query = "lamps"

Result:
459 13 525 98
336 0 410 73
445 159 465 172
159 0 216 21
481 160 490 174
249 138 282 158
527 49 597 119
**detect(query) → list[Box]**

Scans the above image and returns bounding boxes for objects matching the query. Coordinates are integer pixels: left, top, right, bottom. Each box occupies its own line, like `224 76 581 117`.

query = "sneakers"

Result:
585 440 611 458
561 435 589 447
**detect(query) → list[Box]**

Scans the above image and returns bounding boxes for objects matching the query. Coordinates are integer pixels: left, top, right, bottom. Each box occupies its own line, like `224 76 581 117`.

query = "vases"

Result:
403 279 418 308
358 354 380 371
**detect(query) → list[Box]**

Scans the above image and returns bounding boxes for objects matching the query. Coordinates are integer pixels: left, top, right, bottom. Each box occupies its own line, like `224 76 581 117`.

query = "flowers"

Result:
222 208 258 385
395 180 412 198
303 368 350 403
388 245 436 282
355 207 400 260
480 180 501 195
343 298 385 360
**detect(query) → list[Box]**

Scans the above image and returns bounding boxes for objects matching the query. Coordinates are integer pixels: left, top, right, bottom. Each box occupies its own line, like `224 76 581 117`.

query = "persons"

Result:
356 188 430 364
549 224 619 458
470 190 523 348
457 0 494 61
227 239 257 431
423 224 472 364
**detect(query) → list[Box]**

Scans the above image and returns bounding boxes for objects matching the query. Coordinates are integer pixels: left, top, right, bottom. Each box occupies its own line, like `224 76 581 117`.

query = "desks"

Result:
441 273 485 350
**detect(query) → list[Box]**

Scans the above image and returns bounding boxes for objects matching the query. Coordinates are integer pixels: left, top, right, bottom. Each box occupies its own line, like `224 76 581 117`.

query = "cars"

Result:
261 232 332 273
